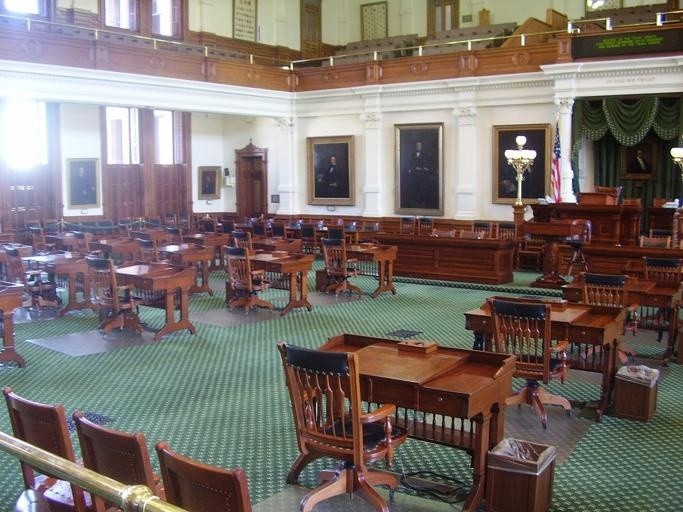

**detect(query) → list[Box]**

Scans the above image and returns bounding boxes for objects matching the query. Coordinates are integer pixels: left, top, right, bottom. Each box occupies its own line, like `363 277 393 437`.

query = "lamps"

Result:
669 147 683 183
504 135 537 202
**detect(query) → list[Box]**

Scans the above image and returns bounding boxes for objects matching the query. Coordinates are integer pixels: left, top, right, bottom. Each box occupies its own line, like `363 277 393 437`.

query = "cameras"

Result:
566 239 584 249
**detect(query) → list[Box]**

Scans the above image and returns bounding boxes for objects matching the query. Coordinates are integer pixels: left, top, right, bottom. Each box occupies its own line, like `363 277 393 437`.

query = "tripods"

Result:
565 250 589 276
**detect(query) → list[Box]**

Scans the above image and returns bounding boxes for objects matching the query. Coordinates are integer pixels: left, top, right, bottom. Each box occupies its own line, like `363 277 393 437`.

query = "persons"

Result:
204 175 214 194
628 148 650 174
317 155 344 197
72 166 94 204
408 141 435 207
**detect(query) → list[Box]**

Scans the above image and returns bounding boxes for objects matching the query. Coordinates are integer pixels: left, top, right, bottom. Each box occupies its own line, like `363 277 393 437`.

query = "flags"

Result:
550 121 561 204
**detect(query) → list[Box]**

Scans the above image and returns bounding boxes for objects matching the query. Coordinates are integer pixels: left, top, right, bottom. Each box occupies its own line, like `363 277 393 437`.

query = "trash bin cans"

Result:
614 365 660 422
486 437 557 512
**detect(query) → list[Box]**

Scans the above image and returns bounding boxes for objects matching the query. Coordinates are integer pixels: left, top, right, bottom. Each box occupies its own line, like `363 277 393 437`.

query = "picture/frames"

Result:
491 122 552 206
393 121 446 217
198 166 222 201
65 158 101 209
619 136 658 181
305 134 357 207
